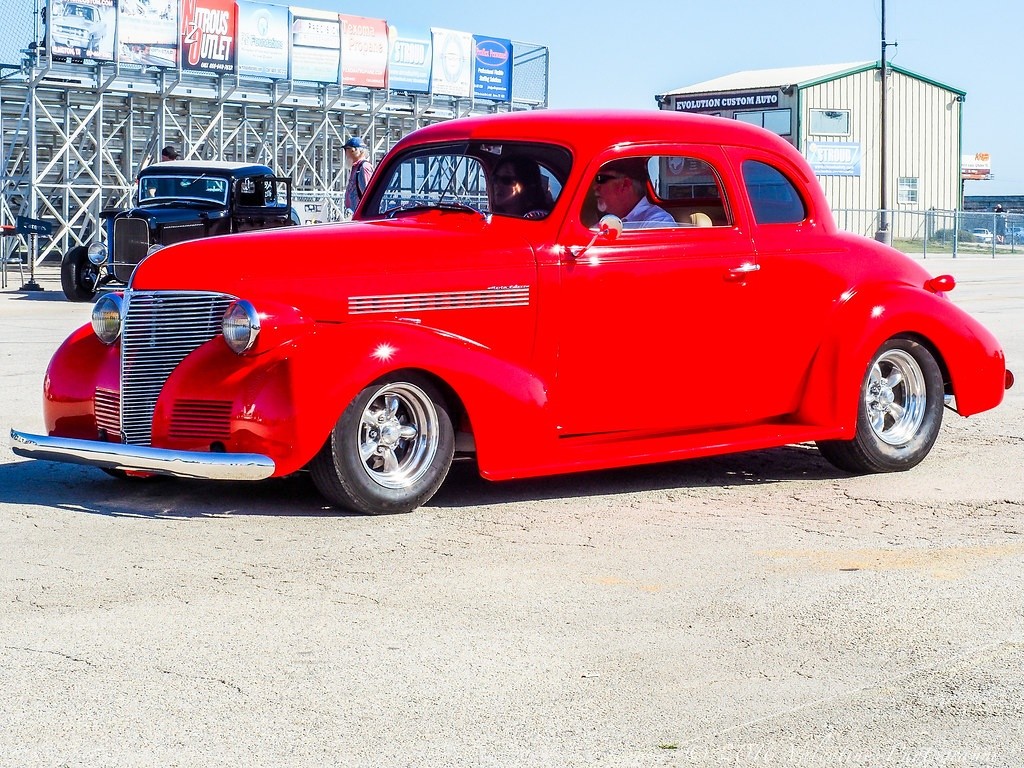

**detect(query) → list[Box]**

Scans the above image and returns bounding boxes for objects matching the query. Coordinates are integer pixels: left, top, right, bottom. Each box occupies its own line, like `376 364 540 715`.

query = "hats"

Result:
601 157 651 181
162 145 181 160
340 136 368 148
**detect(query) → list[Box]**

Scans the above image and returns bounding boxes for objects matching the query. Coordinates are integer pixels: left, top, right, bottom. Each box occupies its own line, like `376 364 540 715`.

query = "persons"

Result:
995 204 1005 244
342 137 372 213
593 157 679 228
490 153 554 216
147 146 179 197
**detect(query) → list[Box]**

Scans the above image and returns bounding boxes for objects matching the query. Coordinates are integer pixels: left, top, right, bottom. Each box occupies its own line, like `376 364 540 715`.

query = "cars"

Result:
52 2 108 54
60 160 303 304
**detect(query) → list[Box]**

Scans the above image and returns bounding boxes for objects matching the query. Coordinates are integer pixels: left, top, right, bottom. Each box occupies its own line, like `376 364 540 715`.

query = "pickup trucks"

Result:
971 227 994 243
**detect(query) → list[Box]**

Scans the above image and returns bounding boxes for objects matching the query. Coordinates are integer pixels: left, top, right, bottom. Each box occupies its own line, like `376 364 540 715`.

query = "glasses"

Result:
594 172 625 185
492 174 522 186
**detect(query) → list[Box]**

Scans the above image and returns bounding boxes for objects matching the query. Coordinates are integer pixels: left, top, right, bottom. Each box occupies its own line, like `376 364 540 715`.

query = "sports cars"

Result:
10 108 1015 517
